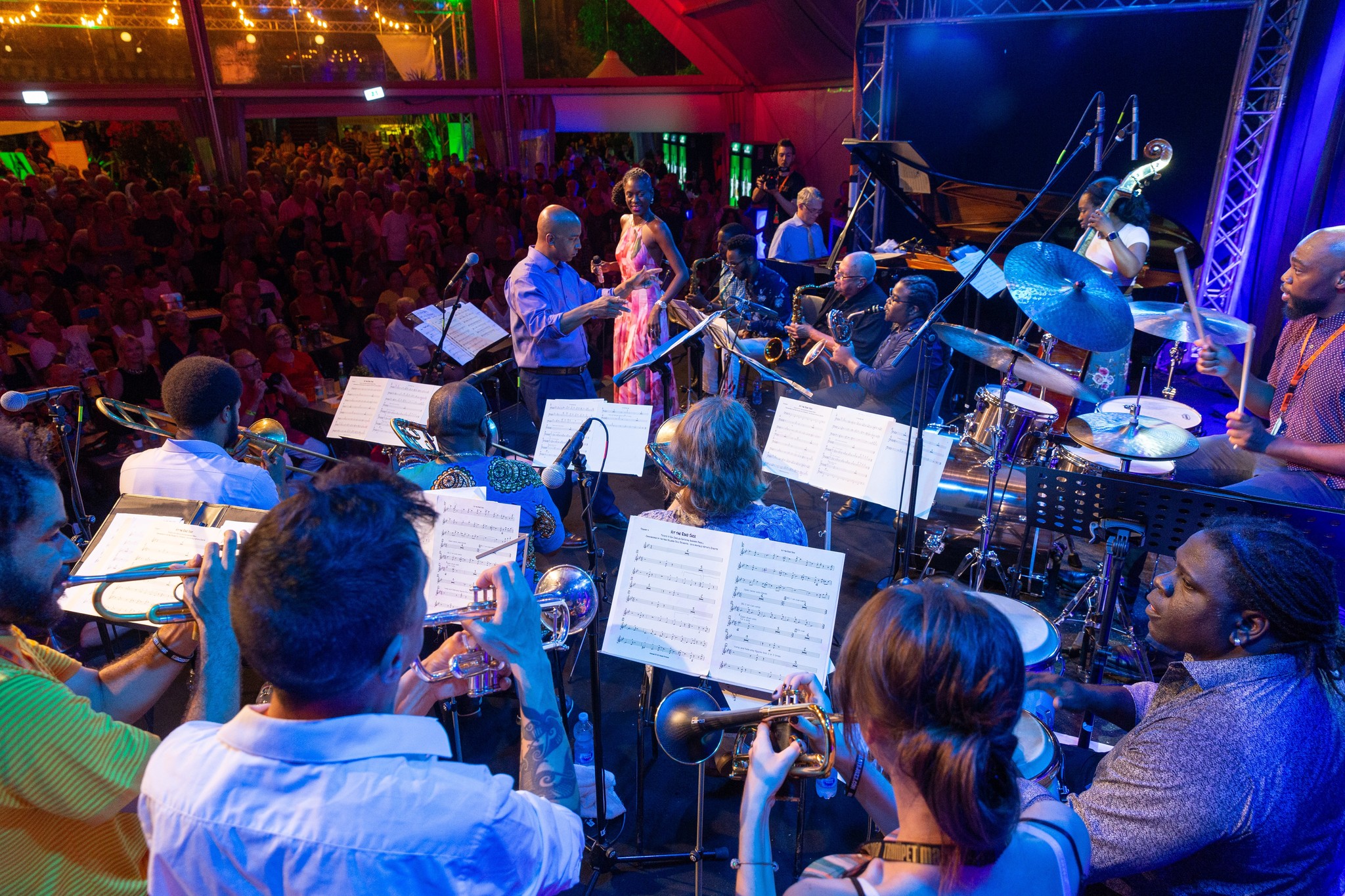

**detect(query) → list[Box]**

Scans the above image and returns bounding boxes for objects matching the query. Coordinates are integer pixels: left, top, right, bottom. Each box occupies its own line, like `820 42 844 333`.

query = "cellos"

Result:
1021 137 1173 435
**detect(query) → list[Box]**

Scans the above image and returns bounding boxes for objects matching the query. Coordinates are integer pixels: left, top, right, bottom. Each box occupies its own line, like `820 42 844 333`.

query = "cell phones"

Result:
259 292 276 302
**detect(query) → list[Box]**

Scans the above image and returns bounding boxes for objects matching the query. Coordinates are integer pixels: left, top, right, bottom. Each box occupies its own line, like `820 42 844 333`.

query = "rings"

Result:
650 329 654 332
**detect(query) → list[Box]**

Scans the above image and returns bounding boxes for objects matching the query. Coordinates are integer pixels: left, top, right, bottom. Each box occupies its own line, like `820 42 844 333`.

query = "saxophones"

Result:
688 252 722 334
803 304 887 389
764 280 838 366
713 273 750 369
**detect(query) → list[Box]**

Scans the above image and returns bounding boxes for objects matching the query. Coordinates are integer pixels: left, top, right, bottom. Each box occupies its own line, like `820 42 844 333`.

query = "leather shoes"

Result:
560 530 588 549
593 511 628 533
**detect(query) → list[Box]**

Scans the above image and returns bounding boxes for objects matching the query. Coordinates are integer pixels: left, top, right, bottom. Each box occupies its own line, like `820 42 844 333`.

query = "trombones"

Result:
389 418 542 475
95 396 349 480
644 413 690 487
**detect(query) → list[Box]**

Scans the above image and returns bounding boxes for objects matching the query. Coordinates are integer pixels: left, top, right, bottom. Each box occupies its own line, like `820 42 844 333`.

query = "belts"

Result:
520 363 587 376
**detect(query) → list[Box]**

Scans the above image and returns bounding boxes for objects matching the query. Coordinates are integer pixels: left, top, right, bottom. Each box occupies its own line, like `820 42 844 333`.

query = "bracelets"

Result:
655 299 667 310
846 748 865 798
1104 230 1119 241
730 856 779 872
151 629 193 664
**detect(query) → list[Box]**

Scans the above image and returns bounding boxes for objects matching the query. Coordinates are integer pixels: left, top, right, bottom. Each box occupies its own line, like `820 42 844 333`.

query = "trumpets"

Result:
55 543 243 625
409 563 599 699
653 685 861 780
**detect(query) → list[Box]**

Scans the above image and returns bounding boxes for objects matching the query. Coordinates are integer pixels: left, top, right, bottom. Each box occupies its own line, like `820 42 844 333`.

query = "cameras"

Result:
763 171 778 189
264 372 284 395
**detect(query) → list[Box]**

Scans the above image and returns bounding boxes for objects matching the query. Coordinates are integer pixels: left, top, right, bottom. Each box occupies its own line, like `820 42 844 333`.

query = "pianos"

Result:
795 137 1205 397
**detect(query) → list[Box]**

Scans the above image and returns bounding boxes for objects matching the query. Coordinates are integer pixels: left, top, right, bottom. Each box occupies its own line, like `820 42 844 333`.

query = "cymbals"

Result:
1003 240 1135 353
1127 300 1255 346
1066 412 1201 462
930 322 1102 404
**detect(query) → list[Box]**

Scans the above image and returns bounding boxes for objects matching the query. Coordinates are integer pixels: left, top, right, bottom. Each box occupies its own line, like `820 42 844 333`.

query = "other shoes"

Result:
1099 640 1183 683
456 694 482 717
515 693 573 732
679 378 701 393
1055 568 1139 614
833 497 861 521
680 397 705 411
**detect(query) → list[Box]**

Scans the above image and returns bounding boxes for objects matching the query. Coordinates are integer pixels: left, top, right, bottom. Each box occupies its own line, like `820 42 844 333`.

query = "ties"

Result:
803 225 816 258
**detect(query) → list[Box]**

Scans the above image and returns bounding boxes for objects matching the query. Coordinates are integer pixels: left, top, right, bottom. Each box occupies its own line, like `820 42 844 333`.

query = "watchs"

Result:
705 301 713 312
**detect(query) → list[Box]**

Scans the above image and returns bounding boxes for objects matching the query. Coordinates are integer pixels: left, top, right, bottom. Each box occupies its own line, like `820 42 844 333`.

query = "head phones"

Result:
770 143 778 162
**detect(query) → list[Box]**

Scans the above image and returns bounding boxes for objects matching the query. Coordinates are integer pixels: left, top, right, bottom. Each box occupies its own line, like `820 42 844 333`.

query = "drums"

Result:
893 422 1057 602
1009 707 1064 802
1044 433 1176 521
959 384 1059 469
960 591 1063 724
1093 395 1205 439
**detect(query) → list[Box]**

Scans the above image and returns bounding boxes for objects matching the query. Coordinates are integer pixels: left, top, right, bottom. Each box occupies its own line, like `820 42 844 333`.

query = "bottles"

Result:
816 764 838 799
573 712 594 767
313 371 324 400
322 332 333 340
297 324 308 351
338 362 348 394
149 433 161 449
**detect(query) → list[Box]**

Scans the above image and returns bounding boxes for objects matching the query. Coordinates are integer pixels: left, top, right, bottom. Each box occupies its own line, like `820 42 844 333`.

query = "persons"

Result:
392 381 574 727
590 167 688 467
678 222 745 391
751 139 805 260
629 392 832 803
119 356 288 509
0 98 755 519
502 205 629 549
1072 178 1151 446
137 456 584 896
1057 224 1345 604
799 275 952 522
728 580 1089 895
768 186 828 262
0 414 250 896
770 251 893 404
1022 512 1345 895
679 234 791 412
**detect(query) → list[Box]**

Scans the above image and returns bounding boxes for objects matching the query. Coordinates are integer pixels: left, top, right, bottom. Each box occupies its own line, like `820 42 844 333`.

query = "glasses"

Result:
834 265 862 280
235 357 260 371
725 258 747 268
888 288 910 305
484 411 492 420
804 203 823 216
277 333 290 340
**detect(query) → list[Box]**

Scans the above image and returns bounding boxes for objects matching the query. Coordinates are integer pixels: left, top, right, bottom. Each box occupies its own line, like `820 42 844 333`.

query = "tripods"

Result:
942 358 1012 595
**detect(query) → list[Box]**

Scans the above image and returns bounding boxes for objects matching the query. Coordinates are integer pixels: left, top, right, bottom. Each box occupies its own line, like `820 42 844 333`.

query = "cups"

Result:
132 439 143 449
304 385 316 402
324 378 335 395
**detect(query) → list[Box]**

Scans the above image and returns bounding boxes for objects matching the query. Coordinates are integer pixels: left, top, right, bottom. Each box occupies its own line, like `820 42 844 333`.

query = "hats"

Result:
30 338 55 369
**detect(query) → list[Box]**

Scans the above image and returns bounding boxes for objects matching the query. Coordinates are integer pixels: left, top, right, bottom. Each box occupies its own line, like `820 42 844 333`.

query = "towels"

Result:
874 238 902 253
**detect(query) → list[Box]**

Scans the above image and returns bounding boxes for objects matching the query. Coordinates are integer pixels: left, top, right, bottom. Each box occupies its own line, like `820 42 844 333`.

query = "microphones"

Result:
445 252 479 292
540 418 591 489
730 295 778 317
461 358 515 384
0 384 80 411
1131 96 1138 161
592 255 605 283
846 149 862 220
1093 92 1105 172
